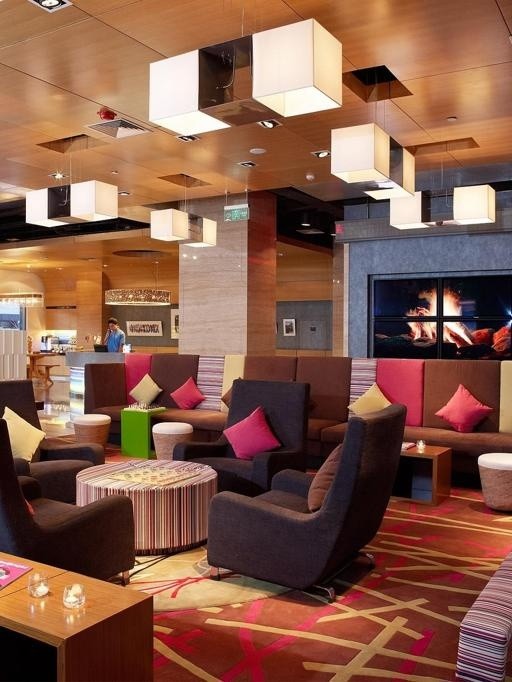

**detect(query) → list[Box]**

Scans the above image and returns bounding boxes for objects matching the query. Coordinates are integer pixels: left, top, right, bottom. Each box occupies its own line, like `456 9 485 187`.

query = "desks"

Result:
26 351 56 366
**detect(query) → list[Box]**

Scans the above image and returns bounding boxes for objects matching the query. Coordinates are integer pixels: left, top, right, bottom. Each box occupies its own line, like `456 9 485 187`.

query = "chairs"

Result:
207 404 406 601
174 380 311 498
0 377 135 588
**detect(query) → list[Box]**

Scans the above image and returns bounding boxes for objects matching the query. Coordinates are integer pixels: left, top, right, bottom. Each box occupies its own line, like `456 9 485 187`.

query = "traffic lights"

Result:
126 372 207 410
433 384 492 432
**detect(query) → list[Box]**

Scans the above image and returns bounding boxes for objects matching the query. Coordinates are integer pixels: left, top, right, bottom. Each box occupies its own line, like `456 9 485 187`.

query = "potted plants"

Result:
283 318 297 337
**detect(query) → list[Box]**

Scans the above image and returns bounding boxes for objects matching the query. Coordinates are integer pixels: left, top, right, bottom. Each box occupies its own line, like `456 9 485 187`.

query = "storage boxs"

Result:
27 363 60 384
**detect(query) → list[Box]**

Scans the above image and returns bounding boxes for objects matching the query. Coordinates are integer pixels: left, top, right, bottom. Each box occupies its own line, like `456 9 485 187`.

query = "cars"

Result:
27 571 50 596
62 582 86 609
416 440 426 453
122 343 132 353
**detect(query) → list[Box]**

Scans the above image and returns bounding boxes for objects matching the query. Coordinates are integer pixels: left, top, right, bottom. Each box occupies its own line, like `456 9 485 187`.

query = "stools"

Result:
476 453 511 511
153 420 192 459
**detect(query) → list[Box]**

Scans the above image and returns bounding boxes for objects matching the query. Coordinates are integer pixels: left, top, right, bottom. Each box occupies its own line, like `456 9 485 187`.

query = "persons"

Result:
101 317 126 353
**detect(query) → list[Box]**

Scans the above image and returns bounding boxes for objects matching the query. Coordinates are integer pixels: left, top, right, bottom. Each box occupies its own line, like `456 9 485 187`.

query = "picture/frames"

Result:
283 318 297 337
26 351 56 366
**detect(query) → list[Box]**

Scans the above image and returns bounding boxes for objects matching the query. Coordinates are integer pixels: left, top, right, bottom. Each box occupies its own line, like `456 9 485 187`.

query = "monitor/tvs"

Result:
0 558 33 591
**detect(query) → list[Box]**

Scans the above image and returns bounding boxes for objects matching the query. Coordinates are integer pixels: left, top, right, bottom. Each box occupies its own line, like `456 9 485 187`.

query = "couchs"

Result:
83 352 511 506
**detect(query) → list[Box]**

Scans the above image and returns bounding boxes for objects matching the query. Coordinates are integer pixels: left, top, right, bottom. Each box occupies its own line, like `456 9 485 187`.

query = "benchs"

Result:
27 363 60 384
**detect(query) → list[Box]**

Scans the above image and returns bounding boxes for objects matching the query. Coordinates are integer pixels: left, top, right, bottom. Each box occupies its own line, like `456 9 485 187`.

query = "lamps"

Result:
241 162 254 167
262 121 275 129
181 135 195 143
331 122 415 200
0 377 135 588
151 209 217 248
26 179 118 228
54 173 64 179
119 191 129 196
390 185 495 229
316 152 328 158
149 18 343 137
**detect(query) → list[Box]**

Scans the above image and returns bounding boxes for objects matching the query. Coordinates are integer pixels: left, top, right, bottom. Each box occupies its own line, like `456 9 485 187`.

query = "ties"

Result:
94 343 108 352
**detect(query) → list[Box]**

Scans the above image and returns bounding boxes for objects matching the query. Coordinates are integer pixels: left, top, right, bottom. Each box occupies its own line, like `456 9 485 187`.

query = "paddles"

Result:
101 542 288 609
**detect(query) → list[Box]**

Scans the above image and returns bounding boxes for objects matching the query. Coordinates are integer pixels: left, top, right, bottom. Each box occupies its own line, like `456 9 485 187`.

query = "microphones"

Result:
153 420 192 459
476 453 511 511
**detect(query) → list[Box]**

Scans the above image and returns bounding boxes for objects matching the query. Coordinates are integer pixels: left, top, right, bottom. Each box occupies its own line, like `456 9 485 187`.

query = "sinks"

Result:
83 352 511 506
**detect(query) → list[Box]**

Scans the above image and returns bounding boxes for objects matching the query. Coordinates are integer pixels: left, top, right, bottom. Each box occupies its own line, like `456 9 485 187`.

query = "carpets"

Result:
101 542 288 609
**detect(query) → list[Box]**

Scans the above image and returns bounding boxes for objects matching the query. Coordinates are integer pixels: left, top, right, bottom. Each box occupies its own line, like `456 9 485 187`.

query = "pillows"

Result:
348 382 392 415
221 384 234 409
126 372 207 410
307 442 344 513
433 384 492 432
223 406 281 460
